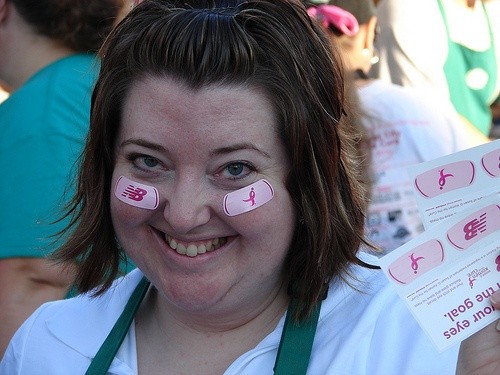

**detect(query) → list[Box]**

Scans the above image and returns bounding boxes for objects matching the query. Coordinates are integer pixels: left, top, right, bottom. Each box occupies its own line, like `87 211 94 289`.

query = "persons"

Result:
2 1 499 375
0 0 121 365
372 1 499 144
304 1 459 172
